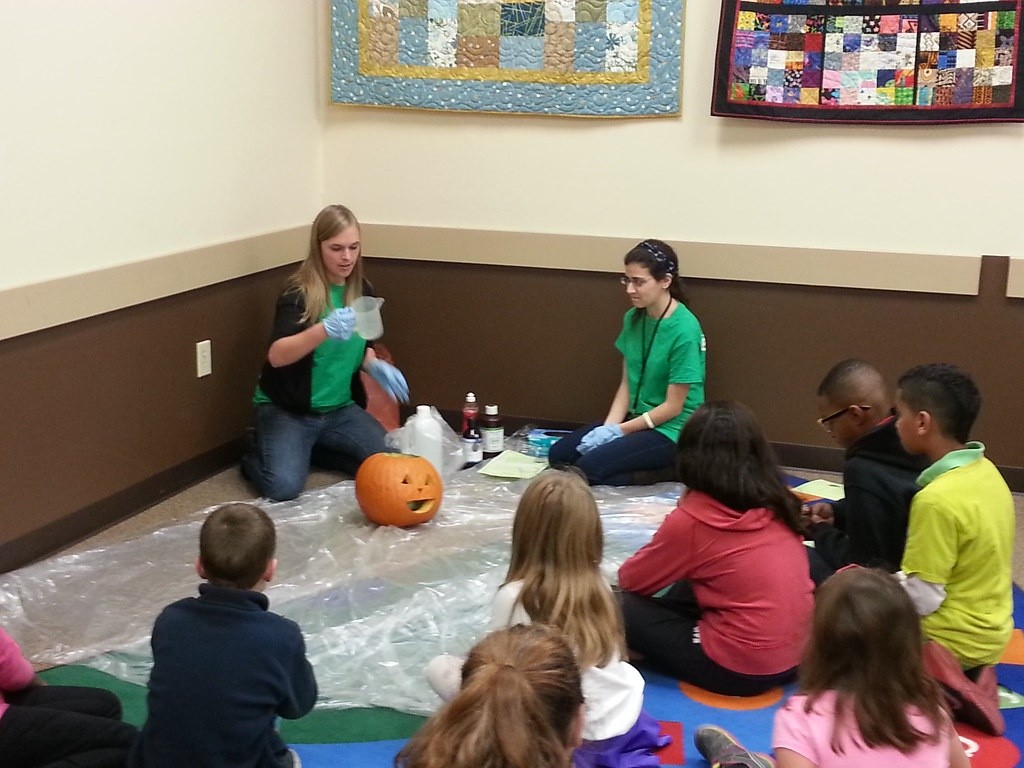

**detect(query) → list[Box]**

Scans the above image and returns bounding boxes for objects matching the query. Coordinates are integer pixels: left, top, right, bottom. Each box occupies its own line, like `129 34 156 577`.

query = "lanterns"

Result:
355 453 443 527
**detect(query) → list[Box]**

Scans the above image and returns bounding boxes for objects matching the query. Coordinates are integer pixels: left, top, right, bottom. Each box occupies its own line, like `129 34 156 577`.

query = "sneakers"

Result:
694 724 778 768
963 664 998 709
923 642 1006 735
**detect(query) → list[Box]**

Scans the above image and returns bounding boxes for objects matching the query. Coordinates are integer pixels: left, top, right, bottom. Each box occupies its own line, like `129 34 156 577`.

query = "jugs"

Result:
346 296 385 340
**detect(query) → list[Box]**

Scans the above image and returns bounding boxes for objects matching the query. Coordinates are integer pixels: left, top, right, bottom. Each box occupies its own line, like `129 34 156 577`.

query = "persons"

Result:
241 204 409 500
424 468 673 768
0 625 137 768
892 362 1014 735
130 502 318 768
694 565 972 768
610 399 817 697
802 359 931 596
393 624 587 768
549 239 706 487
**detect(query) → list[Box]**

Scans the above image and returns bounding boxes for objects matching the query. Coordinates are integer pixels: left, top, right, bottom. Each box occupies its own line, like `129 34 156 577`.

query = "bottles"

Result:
408 404 443 471
460 391 505 465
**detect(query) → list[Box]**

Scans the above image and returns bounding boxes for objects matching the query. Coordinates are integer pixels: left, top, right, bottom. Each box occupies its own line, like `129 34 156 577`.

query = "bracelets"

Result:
643 412 655 429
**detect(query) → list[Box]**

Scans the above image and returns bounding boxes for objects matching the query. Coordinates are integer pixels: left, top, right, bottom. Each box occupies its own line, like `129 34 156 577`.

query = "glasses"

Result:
817 405 871 433
619 274 665 286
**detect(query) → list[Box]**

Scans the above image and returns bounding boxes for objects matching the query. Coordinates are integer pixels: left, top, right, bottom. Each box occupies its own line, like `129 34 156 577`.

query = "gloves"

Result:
575 422 624 455
322 306 357 340
370 359 410 403
